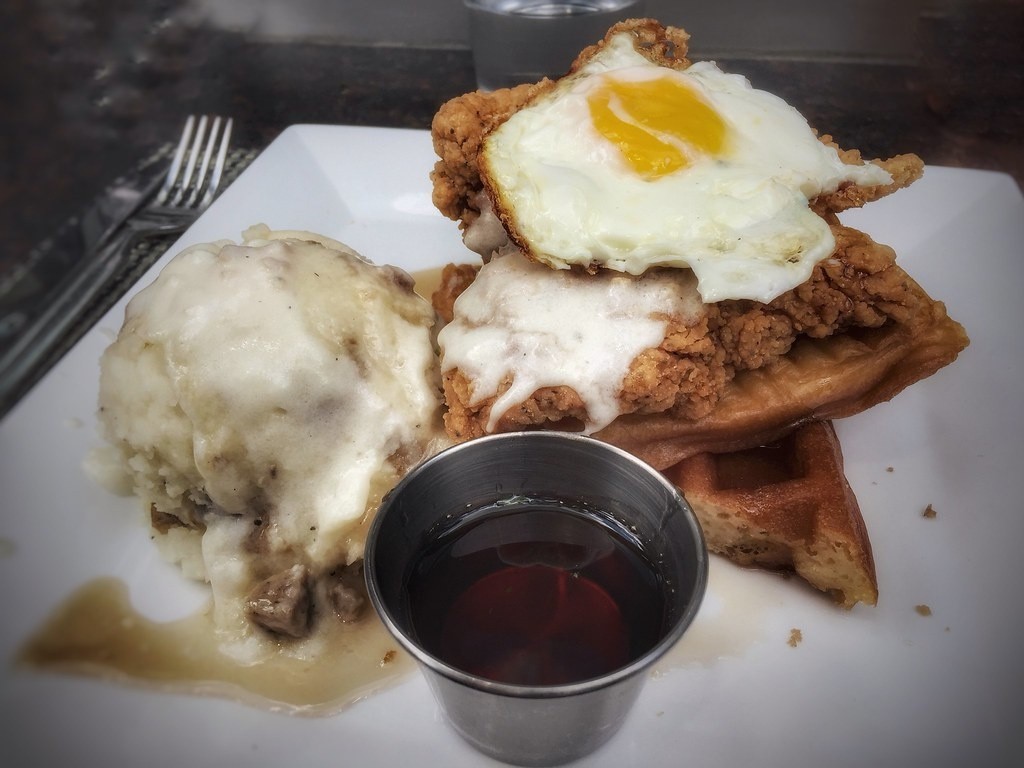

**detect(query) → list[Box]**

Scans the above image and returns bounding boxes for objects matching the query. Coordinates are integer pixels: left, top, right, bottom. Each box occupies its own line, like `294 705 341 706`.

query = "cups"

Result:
464 0 649 104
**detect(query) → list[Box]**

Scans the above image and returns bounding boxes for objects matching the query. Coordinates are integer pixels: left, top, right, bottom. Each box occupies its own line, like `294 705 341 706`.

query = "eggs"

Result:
477 31 894 305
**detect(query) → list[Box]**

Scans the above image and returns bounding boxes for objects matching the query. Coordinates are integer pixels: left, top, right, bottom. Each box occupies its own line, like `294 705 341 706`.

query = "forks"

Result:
2 115 234 418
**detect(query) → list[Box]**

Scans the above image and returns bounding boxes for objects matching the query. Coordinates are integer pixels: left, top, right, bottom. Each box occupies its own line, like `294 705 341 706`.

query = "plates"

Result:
1 123 1024 767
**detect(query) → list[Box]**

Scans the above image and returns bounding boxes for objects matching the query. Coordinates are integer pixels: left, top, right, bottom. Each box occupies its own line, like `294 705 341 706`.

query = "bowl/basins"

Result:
363 430 708 768
